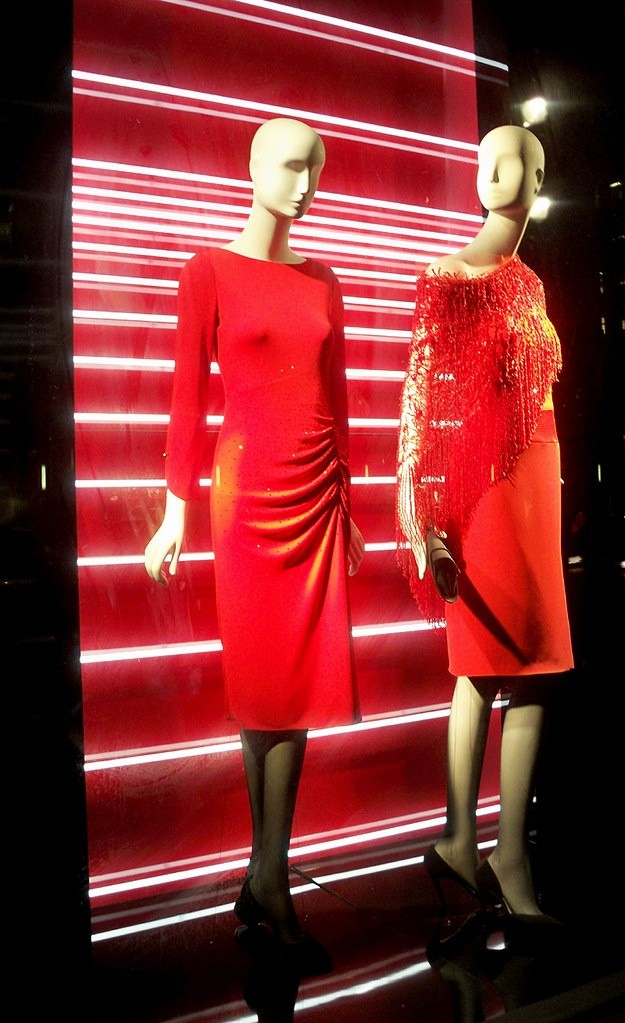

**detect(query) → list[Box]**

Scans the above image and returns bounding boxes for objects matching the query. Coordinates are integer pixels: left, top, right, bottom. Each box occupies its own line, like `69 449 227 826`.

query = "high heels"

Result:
425 902 505 973
233 874 333 971
474 856 569 947
424 845 478 902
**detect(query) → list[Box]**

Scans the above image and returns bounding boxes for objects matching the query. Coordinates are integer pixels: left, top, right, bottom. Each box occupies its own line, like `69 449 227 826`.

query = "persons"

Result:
394 125 576 944
143 118 365 966
